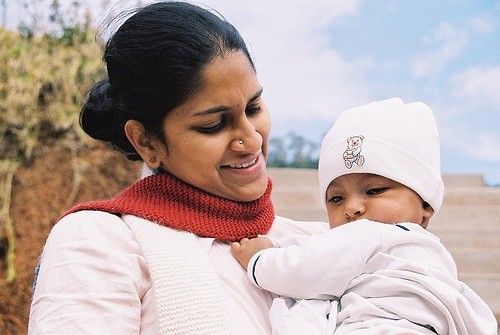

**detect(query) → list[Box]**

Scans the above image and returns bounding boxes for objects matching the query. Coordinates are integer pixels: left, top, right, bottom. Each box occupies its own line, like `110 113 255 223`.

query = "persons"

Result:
230 97 458 335
27 1 498 335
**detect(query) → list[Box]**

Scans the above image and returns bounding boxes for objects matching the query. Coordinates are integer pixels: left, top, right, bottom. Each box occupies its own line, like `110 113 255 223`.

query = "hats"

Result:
318 98 444 216
